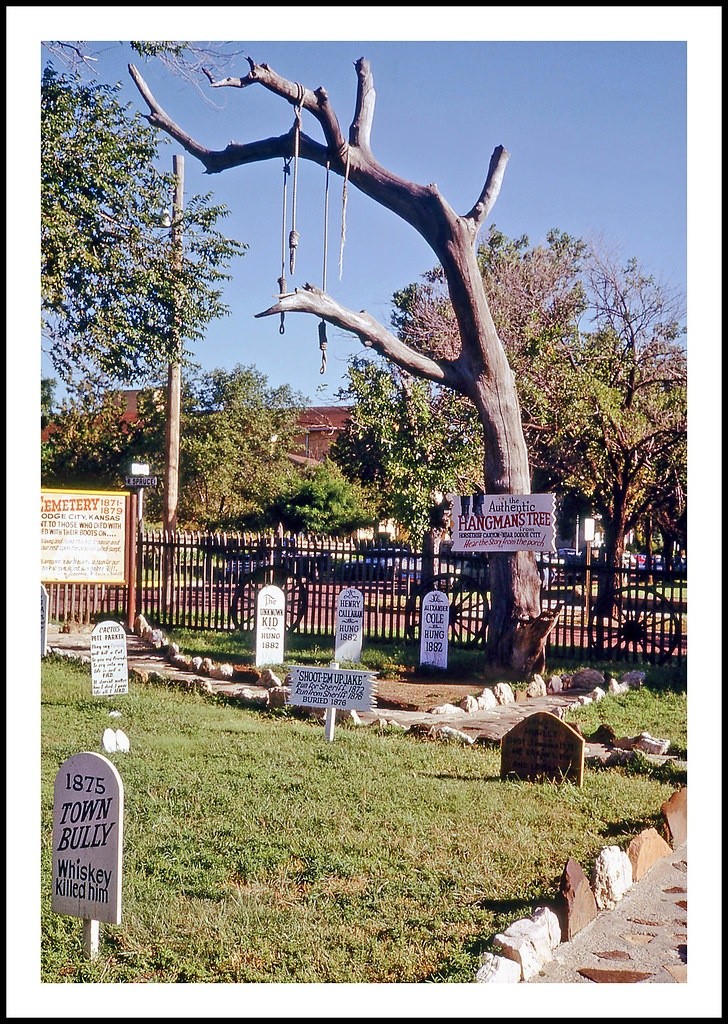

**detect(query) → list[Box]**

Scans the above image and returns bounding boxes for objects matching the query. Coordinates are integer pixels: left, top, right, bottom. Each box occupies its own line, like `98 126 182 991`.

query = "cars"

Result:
345 542 423 582
548 548 577 559
625 552 686 564
457 558 487 583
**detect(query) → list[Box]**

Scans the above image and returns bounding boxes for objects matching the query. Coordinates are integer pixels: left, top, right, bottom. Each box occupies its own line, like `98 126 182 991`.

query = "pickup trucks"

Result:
227 537 334 579
